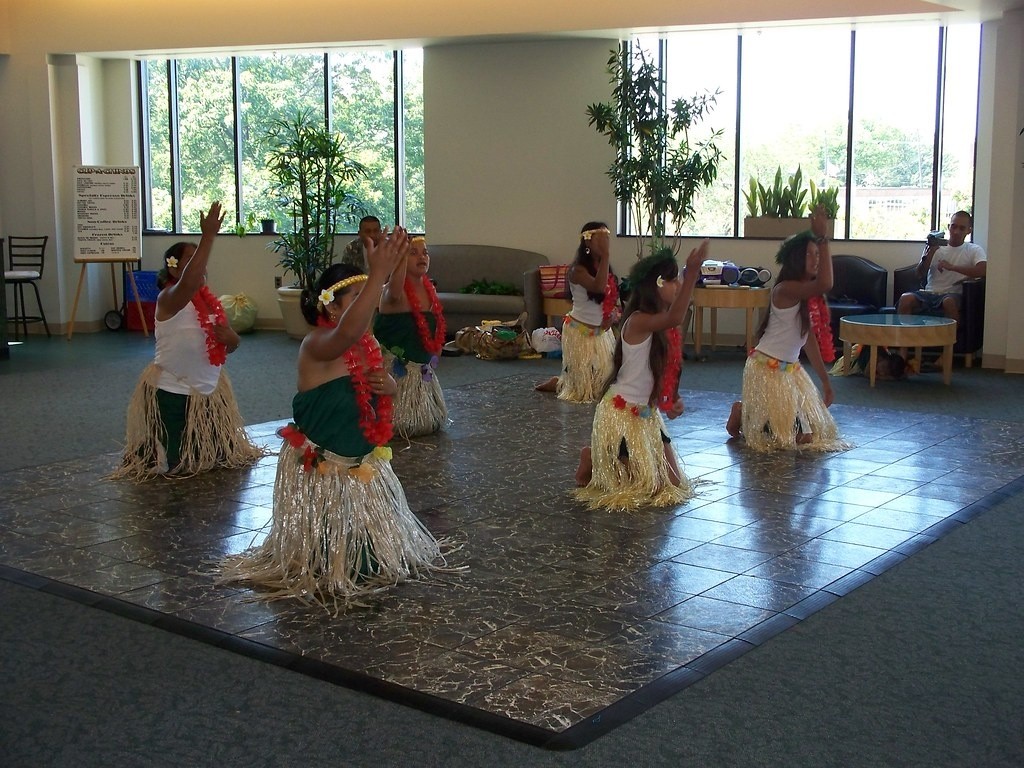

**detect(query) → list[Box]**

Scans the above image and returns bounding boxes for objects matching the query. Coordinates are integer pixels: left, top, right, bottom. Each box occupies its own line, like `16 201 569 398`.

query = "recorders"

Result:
684 260 740 286
737 266 772 286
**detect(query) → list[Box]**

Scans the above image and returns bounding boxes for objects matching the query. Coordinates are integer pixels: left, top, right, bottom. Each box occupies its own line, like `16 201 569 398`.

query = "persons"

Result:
123 201 241 471
726 204 833 449
574 237 709 490
887 211 987 372
343 216 383 272
259 225 410 585
371 231 444 435
531 222 622 395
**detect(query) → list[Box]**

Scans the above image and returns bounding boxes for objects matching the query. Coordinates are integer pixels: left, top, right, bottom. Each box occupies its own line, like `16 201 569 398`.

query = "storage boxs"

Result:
541 351 562 360
123 302 156 333
124 270 162 303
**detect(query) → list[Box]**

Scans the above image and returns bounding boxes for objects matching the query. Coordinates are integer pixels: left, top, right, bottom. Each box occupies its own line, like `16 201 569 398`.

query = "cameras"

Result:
928 230 949 247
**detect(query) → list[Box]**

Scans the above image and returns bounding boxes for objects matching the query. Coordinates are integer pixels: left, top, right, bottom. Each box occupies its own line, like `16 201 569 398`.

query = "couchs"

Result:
425 245 550 338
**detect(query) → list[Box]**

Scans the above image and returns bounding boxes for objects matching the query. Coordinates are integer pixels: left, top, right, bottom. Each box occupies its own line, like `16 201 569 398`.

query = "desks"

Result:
690 288 772 361
839 314 956 388
0 238 10 360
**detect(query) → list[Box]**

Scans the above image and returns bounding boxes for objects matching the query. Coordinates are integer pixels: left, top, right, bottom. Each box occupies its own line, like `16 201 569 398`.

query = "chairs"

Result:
5 236 52 341
878 262 986 368
798 255 887 348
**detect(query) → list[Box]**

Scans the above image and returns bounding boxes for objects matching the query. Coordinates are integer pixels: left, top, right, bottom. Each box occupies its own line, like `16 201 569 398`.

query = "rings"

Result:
379 378 385 388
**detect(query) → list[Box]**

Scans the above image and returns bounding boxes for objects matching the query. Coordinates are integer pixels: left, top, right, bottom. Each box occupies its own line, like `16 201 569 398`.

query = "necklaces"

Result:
317 316 394 443
601 270 617 322
807 297 835 363
405 274 445 353
659 328 682 412
186 285 229 368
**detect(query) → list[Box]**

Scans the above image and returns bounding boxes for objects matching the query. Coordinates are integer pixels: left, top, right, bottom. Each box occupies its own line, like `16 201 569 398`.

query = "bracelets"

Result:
815 234 830 247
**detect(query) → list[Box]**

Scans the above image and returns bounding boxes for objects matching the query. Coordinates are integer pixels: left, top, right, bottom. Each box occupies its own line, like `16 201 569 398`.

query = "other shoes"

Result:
920 362 941 373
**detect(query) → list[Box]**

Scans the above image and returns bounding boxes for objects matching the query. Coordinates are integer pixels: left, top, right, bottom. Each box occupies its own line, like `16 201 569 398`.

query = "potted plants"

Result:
235 209 283 238
740 162 840 238
254 106 371 343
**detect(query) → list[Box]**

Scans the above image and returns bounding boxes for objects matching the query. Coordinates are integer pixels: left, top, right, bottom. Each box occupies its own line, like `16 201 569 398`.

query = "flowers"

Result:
275 421 394 484
166 256 178 268
318 290 335 306
748 347 802 372
563 315 605 338
582 230 592 241
613 393 651 419
389 346 438 383
656 275 665 288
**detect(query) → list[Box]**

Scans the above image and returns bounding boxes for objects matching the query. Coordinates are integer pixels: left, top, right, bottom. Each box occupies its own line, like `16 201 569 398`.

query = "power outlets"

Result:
275 277 282 288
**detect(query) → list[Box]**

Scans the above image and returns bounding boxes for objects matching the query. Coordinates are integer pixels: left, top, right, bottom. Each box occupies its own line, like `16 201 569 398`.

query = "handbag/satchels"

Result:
454 311 562 361
536 264 570 298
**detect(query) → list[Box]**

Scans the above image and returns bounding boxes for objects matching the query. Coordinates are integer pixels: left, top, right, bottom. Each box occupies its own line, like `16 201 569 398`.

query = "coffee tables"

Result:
542 298 573 328
